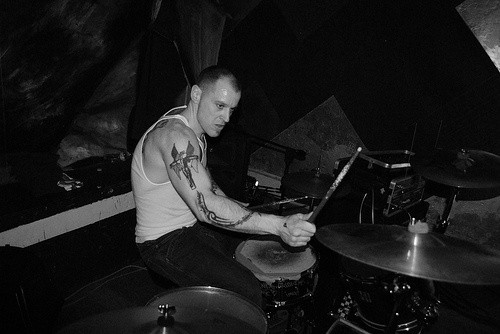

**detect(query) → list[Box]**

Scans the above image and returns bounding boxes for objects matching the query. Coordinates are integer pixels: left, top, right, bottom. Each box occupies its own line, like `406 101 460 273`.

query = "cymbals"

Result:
316 222 500 288
56 305 263 334
281 170 351 200
408 147 500 190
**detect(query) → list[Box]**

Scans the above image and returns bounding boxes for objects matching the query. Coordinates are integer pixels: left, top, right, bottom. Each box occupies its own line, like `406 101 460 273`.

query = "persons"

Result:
131 64 317 293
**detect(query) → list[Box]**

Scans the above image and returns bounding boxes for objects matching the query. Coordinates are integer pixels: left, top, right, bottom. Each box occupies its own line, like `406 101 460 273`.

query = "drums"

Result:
351 267 437 332
223 202 321 286
140 284 269 333
